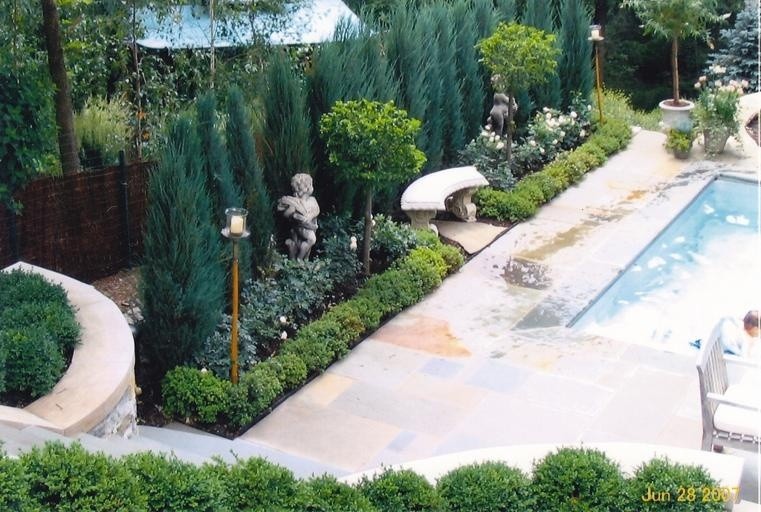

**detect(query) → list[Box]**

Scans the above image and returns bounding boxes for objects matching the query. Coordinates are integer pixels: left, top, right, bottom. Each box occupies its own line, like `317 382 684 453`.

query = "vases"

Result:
703 121 733 155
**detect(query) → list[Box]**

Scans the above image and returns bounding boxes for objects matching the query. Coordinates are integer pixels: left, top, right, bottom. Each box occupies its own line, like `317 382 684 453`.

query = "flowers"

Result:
692 63 750 133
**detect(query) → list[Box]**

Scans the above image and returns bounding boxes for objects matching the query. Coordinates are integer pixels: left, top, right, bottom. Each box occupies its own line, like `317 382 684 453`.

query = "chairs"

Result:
695 312 760 456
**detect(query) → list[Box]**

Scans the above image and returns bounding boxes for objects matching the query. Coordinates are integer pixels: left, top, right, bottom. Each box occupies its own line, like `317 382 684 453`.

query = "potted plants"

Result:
620 0 731 135
664 127 697 158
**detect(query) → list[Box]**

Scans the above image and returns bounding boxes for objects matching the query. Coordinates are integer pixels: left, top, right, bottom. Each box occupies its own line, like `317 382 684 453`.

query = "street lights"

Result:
586 24 608 129
219 207 252 387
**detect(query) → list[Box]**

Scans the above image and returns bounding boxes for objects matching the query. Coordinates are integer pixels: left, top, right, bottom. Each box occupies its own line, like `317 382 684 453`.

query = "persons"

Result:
284 173 319 264
743 310 761 358
490 74 515 137
